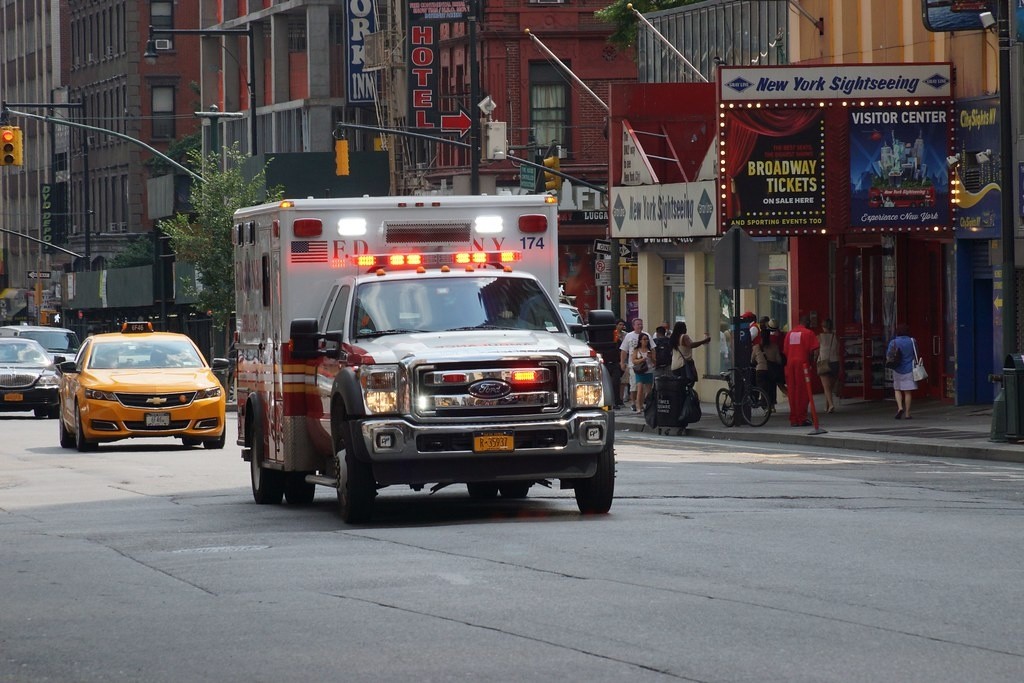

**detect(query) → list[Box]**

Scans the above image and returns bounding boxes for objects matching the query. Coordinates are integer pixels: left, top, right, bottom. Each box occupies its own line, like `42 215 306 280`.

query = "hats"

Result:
740 311 752 318
768 319 779 328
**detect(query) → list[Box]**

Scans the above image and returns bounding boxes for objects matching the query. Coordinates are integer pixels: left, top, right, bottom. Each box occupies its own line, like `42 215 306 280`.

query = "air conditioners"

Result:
87 136 94 145
109 134 113 141
416 163 428 177
530 0 564 4
88 53 94 62
72 225 76 233
155 39 170 49
119 222 127 232
106 46 113 55
76 57 79 65
539 144 568 159
110 223 117 232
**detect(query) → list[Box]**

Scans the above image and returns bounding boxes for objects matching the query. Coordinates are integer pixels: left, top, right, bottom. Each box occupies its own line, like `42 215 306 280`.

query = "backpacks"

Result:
655 339 672 368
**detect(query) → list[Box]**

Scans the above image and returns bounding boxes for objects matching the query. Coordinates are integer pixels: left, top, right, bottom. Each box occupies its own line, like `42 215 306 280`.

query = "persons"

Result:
719 322 734 371
653 321 672 338
888 323 919 419
740 311 786 412
783 317 820 427
106 348 119 368
814 319 839 415
653 326 672 389
616 318 628 341
496 296 514 318
668 322 711 376
632 334 653 414
620 318 657 410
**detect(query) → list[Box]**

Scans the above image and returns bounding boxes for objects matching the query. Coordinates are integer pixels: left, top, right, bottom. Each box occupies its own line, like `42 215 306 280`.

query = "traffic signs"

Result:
26 270 51 279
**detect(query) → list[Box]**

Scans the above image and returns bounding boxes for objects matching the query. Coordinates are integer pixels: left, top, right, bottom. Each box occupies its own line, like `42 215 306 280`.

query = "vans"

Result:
0 325 82 373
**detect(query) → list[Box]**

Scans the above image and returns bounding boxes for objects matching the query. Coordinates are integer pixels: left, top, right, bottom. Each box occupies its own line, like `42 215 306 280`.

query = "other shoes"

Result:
799 419 812 426
828 406 834 414
641 407 644 412
763 408 776 416
791 424 799 427
630 405 636 411
636 410 641 414
824 407 827 412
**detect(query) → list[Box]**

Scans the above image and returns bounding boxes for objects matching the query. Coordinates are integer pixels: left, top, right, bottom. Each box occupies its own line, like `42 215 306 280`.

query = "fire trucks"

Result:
231 193 620 523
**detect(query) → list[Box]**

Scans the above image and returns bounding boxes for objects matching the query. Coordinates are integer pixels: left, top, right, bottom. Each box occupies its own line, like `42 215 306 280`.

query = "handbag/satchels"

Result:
767 361 784 380
885 340 900 370
620 367 630 385
685 360 698 382
911 338 928 382
633 348 648 374
816 359 831 375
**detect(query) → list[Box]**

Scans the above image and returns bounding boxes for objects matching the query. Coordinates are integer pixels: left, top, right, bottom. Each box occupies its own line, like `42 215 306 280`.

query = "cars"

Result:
0 337 67 420
57 322 231 452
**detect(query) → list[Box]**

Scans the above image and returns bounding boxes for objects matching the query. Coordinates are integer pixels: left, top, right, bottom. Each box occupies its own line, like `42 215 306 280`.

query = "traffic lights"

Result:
78 311 83 318
2 129 15 164
542 156 557 192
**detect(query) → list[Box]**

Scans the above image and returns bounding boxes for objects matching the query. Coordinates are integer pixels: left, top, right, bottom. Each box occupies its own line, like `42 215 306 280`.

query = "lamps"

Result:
945 153 960 167
976 149 992 164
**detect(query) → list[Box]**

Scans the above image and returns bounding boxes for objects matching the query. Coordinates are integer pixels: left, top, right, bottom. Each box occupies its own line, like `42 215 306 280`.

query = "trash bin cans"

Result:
1003 353 1024 442
211 356 230 403
654 374 690 428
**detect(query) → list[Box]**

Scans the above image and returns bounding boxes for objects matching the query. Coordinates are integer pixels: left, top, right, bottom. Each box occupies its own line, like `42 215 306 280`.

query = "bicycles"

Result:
715 365 772 427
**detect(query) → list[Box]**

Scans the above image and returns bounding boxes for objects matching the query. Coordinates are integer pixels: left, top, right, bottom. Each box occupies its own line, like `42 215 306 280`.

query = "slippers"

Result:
895 409 904 419
905 415 912 419
